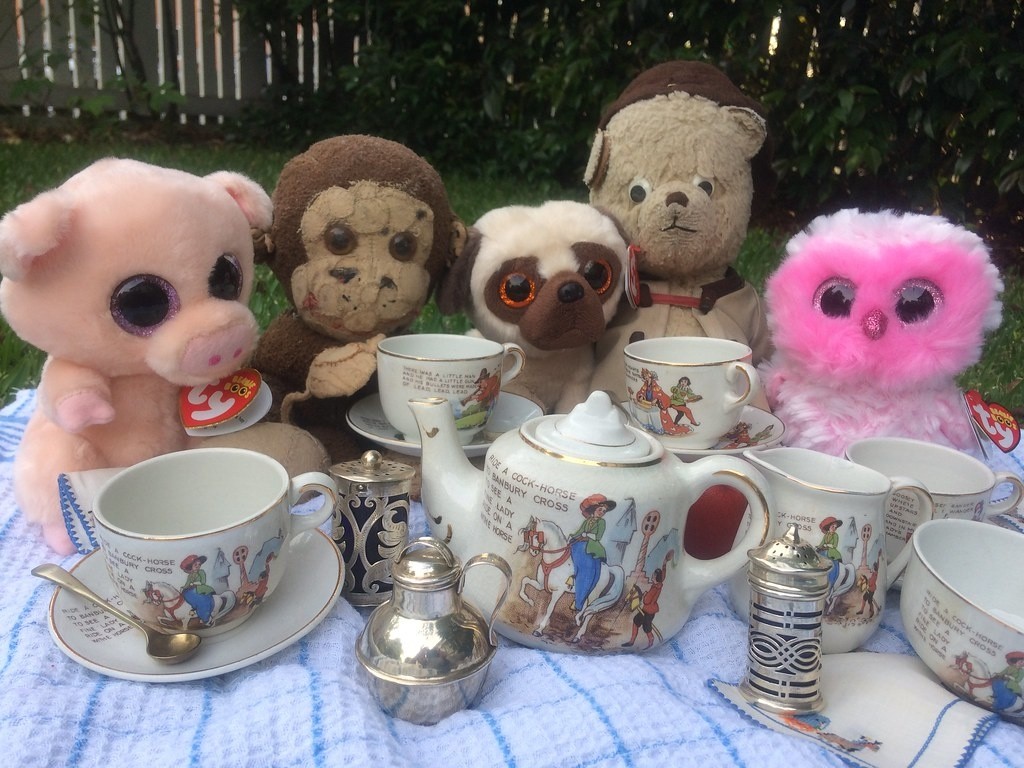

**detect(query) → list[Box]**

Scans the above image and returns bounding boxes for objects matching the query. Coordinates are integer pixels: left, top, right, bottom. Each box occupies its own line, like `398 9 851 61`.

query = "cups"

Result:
899 517 1023 721
622 335 760 451
843 437 1024 589
91 447 340 647
375 332 527 446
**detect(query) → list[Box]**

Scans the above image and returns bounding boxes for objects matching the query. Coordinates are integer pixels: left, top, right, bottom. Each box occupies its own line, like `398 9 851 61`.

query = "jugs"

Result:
736 447 936 655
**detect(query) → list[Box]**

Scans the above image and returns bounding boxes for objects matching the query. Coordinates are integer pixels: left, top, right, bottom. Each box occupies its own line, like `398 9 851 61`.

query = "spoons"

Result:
32 563 202 665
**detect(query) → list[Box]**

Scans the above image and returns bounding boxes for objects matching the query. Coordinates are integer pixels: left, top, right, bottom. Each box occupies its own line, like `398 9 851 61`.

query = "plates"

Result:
45 527 345 684
345 390 544 459
622 400 788 456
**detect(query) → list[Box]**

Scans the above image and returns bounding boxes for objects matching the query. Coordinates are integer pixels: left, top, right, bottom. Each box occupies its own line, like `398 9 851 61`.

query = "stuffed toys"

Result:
199 134 468 503
755 208 1004 460
436 199 639 473
0 156 275 557
588 61 774 403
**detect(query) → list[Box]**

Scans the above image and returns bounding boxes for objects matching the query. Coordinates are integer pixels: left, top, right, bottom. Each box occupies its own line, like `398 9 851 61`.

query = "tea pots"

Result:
405 389 778 656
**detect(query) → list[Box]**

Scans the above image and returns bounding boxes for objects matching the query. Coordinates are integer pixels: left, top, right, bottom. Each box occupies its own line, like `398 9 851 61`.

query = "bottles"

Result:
355 536 513 728
740 523 836 718
327 450 416 607
